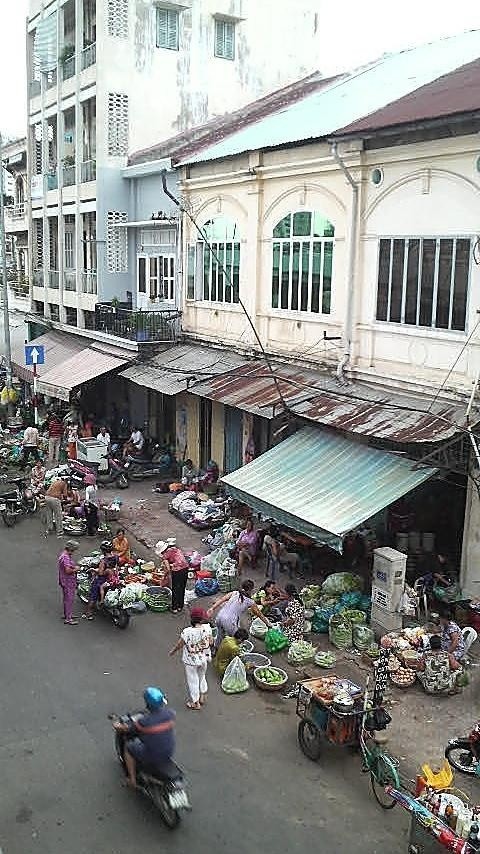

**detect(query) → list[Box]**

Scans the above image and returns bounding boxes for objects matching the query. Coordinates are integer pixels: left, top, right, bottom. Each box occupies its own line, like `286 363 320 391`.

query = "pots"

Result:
333 694 354 713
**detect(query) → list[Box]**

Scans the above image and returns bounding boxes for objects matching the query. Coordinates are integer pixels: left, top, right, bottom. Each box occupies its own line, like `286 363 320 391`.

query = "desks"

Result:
440 594 472 620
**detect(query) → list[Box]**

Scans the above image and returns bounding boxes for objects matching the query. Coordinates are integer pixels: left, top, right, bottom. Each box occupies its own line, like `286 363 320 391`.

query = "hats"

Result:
155 540 168 555
85 474 97 485
191 607 205 619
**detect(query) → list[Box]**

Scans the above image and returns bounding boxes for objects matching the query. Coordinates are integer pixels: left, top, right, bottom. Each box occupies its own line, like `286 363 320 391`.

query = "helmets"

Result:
143 685 165 708
100 541 113 551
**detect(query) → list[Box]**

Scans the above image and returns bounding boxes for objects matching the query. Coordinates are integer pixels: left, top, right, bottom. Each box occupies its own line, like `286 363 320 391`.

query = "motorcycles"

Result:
67 443 129 489
0 476 40 527
107 706 193 829
76 555 131 629
123 441 174 482
441 720 480 776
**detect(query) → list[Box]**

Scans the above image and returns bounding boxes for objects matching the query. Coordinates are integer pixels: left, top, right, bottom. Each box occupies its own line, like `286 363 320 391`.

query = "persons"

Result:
57 520 370 708
1 397 240 540
112 686 176 787
387 498 466 697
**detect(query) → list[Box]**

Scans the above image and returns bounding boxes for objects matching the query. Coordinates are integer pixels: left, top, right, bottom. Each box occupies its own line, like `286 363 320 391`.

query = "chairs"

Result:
461 627 478 656
263 544 291 581
232 529 258 569
404 576 428 621
126 437 153 457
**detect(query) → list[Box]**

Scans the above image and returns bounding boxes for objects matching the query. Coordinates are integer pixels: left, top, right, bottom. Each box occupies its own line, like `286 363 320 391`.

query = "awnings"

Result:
187 360 480 463
221 426 437 555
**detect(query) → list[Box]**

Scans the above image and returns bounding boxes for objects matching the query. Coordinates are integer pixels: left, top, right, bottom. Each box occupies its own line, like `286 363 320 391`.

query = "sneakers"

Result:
170 607 182 614
186 702 202 711
63 613 95 625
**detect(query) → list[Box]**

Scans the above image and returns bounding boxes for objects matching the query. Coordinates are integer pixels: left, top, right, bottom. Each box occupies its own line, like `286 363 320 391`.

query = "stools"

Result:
422 759 453 788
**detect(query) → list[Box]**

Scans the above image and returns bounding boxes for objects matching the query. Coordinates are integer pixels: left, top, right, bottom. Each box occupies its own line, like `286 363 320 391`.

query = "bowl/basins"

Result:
63 523 110 536
237 639 287 690
124 574 146 584
141 564 156 573
148 586 170 612
303 608 315 620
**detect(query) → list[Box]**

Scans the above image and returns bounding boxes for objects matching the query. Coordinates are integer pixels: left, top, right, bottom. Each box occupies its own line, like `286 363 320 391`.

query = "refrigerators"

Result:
76 437 108 475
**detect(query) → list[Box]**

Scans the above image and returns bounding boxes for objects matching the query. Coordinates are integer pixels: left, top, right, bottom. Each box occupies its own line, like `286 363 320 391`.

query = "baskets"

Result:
240 640 290 692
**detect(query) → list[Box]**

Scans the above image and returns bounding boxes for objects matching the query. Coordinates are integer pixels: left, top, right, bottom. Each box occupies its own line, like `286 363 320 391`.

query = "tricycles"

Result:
295 674 402 810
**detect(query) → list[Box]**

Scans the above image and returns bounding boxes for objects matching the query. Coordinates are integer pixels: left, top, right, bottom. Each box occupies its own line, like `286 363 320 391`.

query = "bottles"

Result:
419 784 480 847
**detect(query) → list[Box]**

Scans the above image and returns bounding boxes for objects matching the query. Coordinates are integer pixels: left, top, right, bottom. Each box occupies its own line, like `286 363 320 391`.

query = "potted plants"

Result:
111 294 170 341
